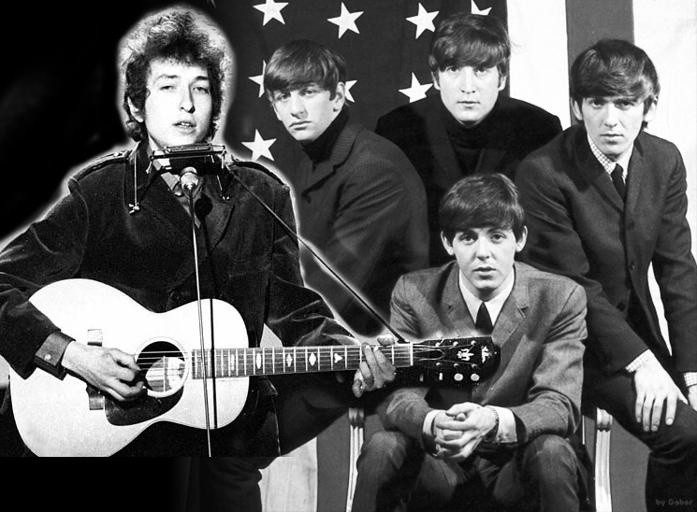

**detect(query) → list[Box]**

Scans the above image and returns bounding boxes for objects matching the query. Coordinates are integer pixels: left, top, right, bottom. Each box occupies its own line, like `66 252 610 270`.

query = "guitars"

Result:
7 277 501 458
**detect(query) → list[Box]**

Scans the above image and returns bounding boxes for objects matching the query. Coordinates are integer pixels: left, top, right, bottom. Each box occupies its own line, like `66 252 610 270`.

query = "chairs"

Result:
346 403 613 512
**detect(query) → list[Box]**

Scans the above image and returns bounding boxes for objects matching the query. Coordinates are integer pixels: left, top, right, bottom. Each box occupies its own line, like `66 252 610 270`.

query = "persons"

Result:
349 171 594 512
516 38 696 511
0 6 397 512
247 39 430 469
373 13 564 269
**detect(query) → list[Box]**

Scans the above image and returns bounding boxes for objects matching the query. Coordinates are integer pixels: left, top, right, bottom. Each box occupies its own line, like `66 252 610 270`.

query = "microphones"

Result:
180 166 201 199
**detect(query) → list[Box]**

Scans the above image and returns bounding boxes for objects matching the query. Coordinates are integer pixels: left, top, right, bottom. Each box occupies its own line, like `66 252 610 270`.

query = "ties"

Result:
472 303 496 339
608 162 630 203
